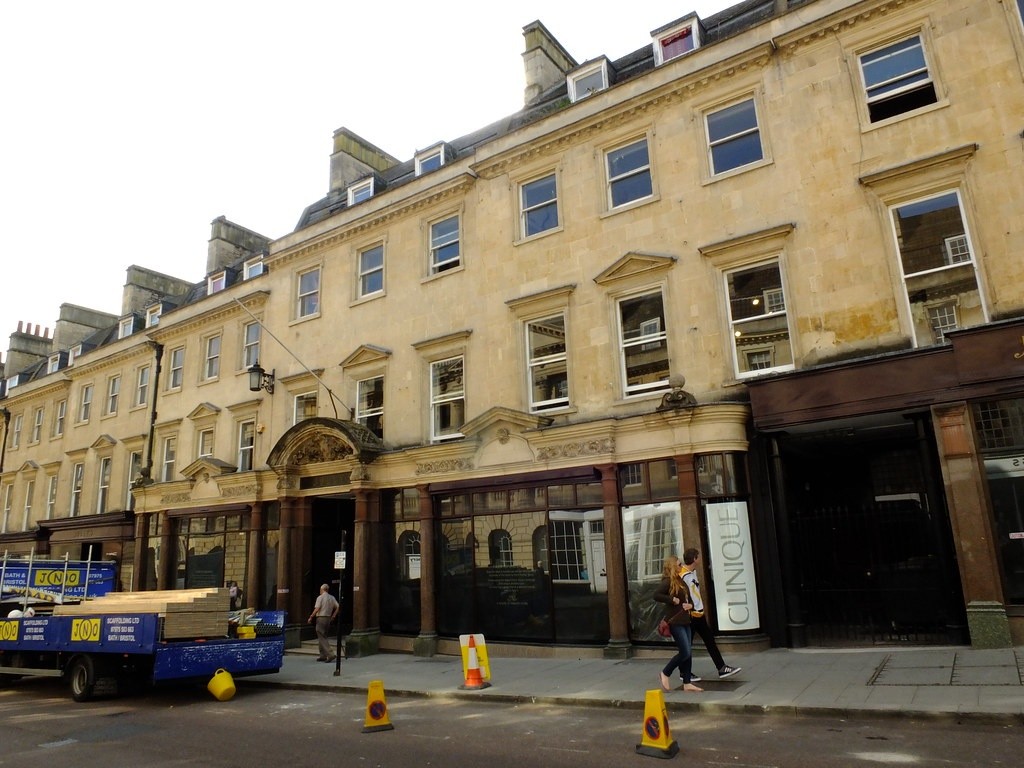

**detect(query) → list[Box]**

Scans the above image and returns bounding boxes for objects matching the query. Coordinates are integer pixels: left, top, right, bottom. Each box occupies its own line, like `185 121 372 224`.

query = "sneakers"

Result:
680 672 702 681
719 665 742 678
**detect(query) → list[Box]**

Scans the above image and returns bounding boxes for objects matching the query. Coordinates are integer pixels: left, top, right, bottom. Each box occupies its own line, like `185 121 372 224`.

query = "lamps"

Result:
248 358 275 396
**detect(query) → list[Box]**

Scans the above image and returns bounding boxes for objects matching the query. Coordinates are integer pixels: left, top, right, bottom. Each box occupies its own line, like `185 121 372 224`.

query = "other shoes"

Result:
315 656 325 662
683 685 703 692
325 655 335 663
659 672 670 691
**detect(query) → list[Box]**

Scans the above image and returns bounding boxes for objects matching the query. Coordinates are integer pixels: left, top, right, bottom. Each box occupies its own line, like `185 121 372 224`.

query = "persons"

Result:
653 556 704 693
229 581 237 611
679 549 741 681
308 584 339 663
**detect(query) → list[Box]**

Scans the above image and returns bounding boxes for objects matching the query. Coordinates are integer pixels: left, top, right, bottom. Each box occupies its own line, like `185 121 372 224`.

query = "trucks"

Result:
0 557 288 703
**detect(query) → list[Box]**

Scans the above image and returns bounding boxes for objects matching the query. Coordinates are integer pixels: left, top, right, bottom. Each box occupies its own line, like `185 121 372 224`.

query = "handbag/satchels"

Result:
658 619 671 638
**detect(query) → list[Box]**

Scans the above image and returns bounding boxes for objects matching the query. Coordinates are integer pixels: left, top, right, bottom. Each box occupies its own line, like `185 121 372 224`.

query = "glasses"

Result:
676 563 682 567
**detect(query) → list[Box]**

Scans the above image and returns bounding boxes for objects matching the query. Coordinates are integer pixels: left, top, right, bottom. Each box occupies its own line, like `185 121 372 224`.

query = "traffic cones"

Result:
459 636 492 690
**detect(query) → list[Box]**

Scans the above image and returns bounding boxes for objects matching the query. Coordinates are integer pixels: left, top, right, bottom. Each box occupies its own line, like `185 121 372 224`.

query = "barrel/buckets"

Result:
207 669 236 701
